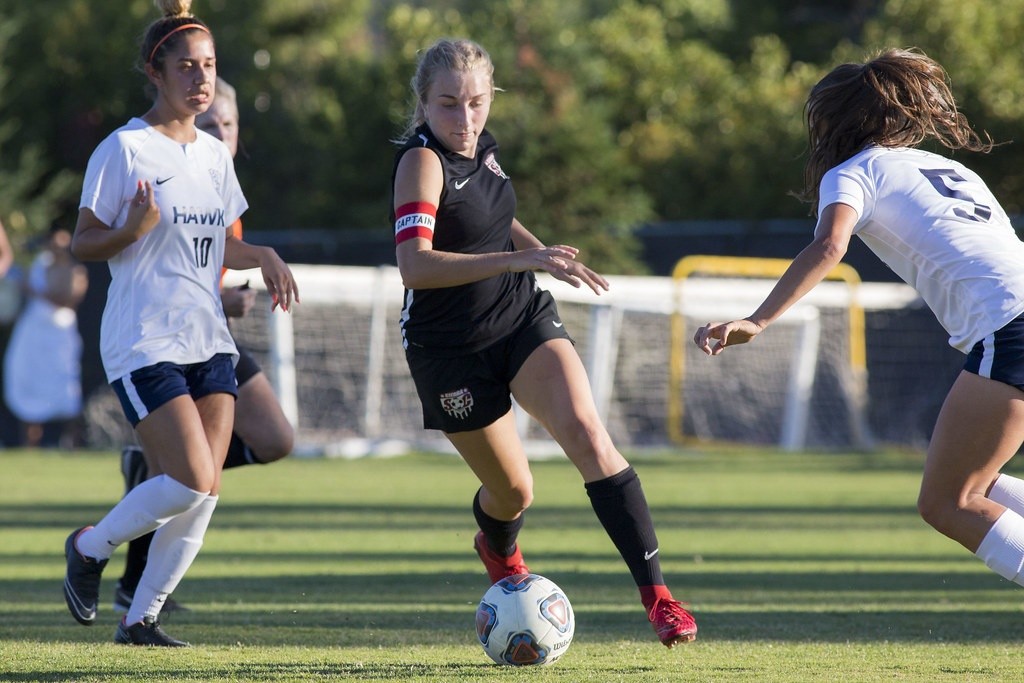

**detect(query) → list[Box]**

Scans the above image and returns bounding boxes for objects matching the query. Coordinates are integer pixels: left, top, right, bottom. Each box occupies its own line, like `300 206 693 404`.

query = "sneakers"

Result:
113 578 191 614
114 615 191 647
121 446 148 495
62 525 110 626
474 531 531 586
639 585 698 649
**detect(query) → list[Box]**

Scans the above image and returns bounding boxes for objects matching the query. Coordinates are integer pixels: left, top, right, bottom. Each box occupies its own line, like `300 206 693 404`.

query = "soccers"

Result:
477 575 576 668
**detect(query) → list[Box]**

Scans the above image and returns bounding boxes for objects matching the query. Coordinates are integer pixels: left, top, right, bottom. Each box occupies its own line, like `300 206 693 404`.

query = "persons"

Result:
693 47 1024 587
0 214 113 449
392 40 698 648
64 0 300 648
